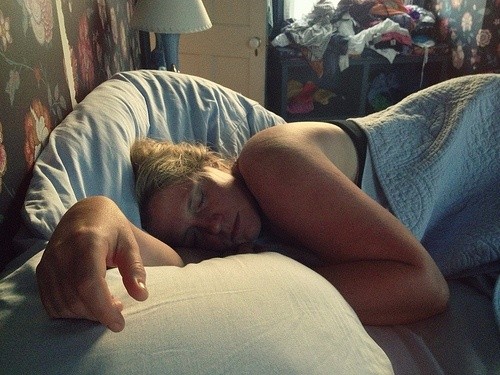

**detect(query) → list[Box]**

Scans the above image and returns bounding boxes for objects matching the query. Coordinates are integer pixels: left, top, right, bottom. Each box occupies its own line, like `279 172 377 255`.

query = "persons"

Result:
35 72 500 333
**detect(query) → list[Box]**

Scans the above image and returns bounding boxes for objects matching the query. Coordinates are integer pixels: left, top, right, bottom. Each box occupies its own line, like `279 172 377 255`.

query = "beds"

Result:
0 70 500 375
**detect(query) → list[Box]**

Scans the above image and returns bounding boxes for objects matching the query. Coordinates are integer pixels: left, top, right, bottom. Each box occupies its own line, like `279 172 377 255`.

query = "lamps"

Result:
129 0 214 74
